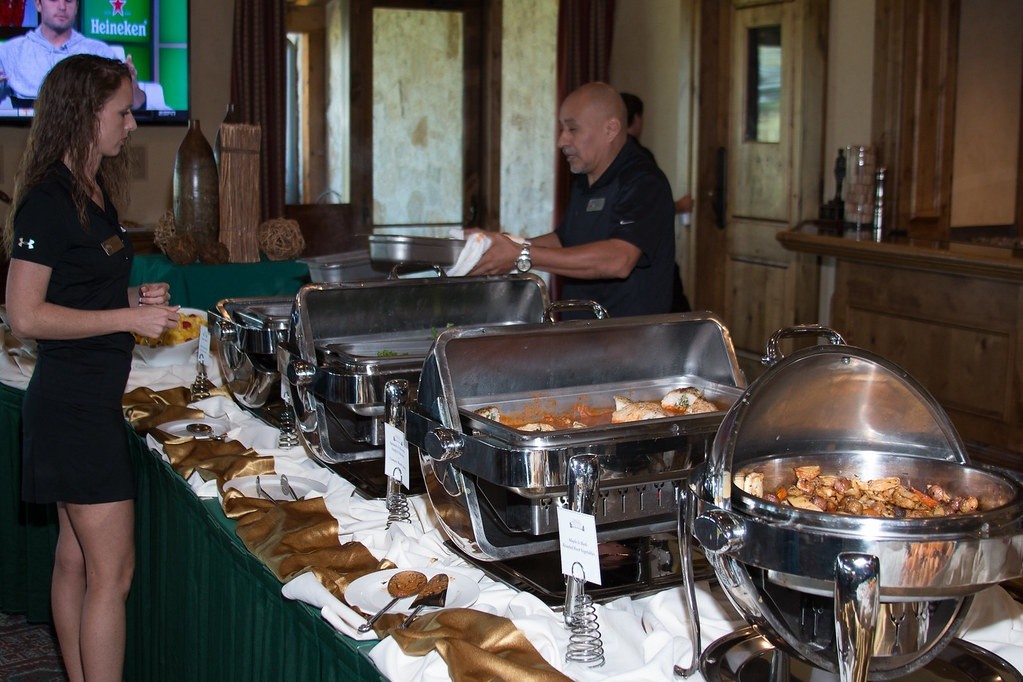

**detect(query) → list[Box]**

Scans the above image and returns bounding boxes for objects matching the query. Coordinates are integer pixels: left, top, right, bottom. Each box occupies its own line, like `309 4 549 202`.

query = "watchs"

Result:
517 244 532 273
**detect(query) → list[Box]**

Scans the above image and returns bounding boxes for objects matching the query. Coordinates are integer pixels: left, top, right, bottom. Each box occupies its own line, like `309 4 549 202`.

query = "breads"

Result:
167 231 229 265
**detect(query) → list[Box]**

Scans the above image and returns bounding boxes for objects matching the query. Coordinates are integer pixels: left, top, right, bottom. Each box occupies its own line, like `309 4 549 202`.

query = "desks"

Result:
0 309 1023 682
132 255 309 312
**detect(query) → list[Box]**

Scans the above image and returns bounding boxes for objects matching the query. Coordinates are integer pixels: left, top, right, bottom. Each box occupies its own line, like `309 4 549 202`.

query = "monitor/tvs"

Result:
0 0 191 127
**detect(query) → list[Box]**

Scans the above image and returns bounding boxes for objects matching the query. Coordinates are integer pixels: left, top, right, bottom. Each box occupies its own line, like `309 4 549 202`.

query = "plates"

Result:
222 475 329 499
155 419 240 440
344 567 480 615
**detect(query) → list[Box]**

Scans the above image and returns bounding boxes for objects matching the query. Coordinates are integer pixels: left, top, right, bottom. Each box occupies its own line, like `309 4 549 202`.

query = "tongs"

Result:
255 474 299 504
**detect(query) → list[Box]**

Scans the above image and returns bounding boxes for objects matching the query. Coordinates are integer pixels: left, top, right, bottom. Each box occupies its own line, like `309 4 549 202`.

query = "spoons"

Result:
358 570 428 633
186 424 225 441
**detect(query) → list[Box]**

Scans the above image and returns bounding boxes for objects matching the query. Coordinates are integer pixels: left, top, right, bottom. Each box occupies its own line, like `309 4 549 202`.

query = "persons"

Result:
465 81 692 321
0 0 147 112
620 91 694 214
4 55 181 681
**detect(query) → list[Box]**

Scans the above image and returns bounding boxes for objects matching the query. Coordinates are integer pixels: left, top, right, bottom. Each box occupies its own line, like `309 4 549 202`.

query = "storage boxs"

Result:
248 303 291 324
332 338 433 362
